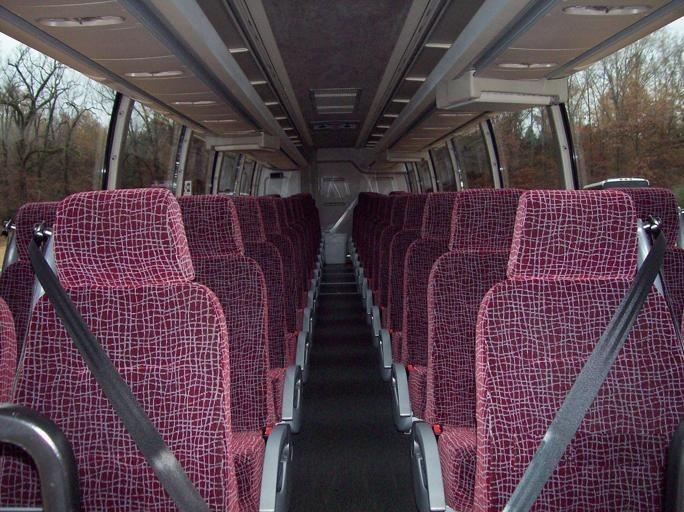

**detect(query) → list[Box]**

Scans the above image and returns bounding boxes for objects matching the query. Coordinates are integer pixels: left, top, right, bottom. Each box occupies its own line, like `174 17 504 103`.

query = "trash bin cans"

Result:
325 234 346 264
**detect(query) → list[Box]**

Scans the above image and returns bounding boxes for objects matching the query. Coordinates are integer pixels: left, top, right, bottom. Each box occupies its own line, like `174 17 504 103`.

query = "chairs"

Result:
0 188 325 507
349 190 684 507
